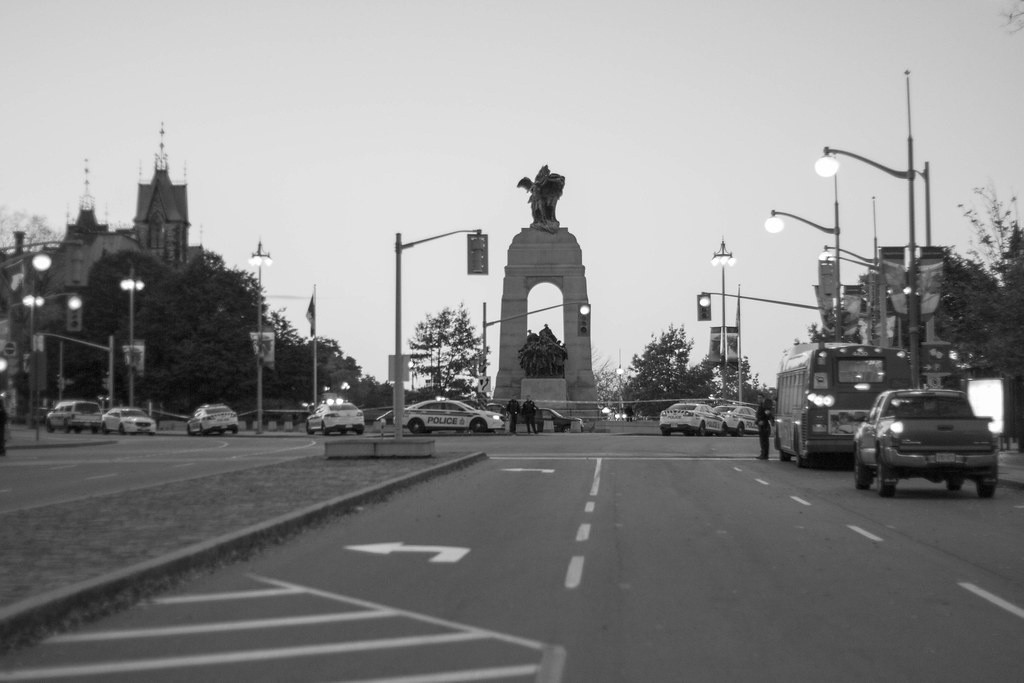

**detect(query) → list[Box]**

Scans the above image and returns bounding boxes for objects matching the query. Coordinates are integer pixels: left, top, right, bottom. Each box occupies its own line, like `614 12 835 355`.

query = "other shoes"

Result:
528 431 531 433
756 454 768 459
535 431 538 434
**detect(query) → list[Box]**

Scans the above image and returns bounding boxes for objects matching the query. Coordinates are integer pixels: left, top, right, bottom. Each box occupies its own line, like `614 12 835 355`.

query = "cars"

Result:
521 408 583 433
393 399 505 434
659 403 771 436
101 406 156 435
187 404 239 435
306 403 364 436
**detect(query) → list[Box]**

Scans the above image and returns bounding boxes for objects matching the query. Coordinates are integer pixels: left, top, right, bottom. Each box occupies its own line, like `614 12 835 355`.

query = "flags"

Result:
306 292 315 337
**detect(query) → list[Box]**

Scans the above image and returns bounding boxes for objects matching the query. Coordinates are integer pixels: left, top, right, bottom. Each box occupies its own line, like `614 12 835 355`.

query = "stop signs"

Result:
3 341 16 356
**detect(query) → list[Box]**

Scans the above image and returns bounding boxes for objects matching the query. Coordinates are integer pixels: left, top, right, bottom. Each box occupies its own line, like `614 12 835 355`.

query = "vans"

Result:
45 401 103 433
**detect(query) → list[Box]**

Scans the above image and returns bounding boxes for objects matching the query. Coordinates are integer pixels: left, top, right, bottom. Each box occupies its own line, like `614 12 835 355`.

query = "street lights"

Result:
711 234 734 404
815 146 922 386
120 275 146 407
819 244 877 346
29 291 82 428
250 241 272 433
766 210 843 341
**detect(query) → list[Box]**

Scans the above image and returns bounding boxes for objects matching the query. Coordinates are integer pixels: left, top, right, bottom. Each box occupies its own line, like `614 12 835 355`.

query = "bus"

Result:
772 341 912 469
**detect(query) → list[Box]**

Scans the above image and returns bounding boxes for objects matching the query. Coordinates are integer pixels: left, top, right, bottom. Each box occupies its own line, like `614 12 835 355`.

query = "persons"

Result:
523 394 538 433
509 394 520 434
625 403 633 422
756 394 771 460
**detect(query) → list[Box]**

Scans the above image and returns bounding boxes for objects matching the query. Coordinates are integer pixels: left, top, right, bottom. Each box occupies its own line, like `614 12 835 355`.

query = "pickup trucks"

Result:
852 388 998 497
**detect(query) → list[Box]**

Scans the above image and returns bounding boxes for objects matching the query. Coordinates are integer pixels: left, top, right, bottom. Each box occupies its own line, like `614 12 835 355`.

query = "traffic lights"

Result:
697 294 711 321
577 303 591 337
478 357 487 373
